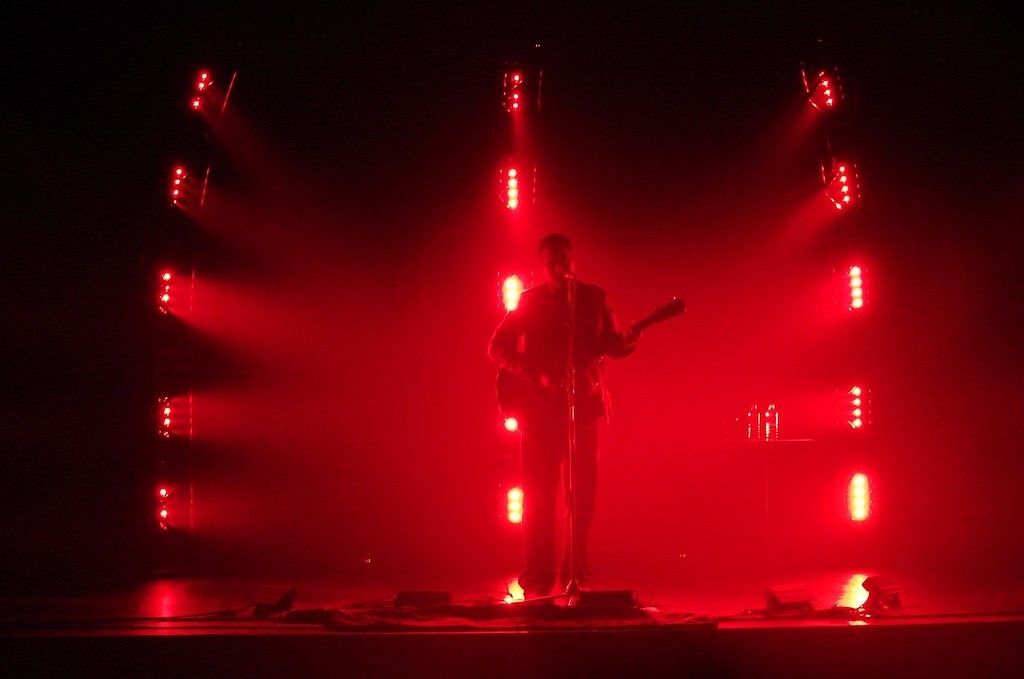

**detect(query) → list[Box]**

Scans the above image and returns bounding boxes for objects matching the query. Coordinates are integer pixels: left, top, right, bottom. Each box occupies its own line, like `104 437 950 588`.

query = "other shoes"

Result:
562 579 592 596
524 590 554 608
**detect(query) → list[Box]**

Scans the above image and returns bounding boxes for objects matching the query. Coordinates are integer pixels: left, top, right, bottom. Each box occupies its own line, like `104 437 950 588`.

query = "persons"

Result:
487 233 641 605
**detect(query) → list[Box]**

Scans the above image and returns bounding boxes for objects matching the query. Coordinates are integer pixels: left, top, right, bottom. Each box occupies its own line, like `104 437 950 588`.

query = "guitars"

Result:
496 294 685 430
